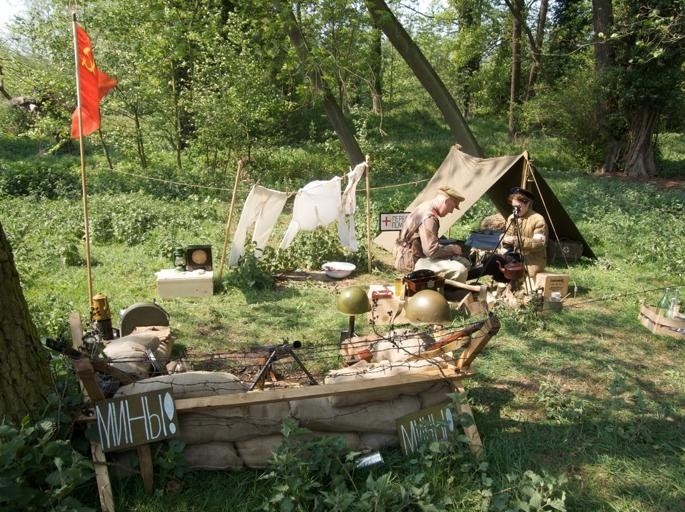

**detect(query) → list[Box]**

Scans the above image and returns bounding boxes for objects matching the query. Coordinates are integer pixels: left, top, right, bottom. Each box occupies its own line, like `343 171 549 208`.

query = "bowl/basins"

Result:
321 261 357 278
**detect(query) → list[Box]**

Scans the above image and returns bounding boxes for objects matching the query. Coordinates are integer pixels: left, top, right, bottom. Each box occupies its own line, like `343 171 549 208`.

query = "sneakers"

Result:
444 290 467 300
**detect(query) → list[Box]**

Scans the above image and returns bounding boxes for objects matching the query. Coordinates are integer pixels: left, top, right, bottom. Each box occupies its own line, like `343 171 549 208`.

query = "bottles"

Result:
656 288 682 317
98 319 113 340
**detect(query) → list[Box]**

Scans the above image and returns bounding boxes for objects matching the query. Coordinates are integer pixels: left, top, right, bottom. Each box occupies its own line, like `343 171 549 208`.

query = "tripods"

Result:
473 219 534 298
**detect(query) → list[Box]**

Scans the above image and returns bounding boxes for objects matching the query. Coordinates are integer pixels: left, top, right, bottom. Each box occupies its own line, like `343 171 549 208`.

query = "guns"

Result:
267 341 300 362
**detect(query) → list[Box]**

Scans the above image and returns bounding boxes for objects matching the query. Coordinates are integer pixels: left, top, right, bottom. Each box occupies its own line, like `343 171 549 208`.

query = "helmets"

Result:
336 286 371 314
406 290 450 323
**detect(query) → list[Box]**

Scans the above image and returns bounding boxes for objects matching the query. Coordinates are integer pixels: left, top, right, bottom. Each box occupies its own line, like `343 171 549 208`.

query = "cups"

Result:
551 292 561 302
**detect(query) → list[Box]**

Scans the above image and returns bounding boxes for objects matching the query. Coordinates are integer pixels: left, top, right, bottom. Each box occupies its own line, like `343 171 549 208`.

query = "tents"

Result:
370 143 600 261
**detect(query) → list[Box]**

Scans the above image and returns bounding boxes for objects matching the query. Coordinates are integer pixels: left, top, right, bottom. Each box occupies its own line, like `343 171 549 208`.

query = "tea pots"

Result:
92 293 111 320
495 253 526 281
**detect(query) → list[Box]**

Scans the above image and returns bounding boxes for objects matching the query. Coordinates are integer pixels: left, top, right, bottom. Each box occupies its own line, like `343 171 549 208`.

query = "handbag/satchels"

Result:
99 326 173 379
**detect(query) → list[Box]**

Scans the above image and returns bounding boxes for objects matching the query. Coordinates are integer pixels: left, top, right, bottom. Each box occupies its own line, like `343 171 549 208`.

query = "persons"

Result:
483 185 551 287
391 186 472 283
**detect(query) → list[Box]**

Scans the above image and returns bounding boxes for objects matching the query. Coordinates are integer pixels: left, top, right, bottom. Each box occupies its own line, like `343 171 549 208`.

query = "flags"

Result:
67 23 116 142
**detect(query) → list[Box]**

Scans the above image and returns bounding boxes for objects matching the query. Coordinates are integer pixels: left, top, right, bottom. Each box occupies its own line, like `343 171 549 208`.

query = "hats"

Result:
440 186 466 210
511 187 536 201
